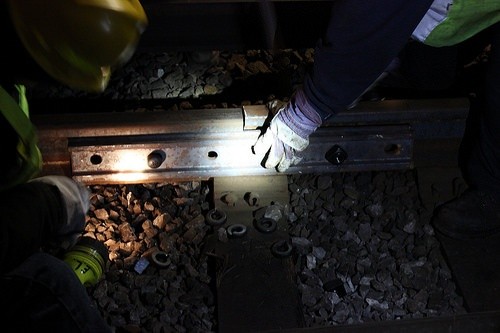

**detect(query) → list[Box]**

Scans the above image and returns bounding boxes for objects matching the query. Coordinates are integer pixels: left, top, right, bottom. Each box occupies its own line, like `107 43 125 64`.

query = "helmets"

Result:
10 0 147 93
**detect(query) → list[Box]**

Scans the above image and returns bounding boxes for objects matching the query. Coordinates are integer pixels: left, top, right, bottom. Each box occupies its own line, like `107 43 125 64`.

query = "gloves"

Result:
29 175 90 235
253 88 322 173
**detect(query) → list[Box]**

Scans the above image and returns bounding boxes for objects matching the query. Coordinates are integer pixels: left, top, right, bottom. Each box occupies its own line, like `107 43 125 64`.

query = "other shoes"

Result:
431 173 500 243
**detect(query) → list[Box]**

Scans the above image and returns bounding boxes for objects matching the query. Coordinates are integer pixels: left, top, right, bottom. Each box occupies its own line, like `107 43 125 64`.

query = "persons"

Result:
0 0 148 333
251 0 499 173
